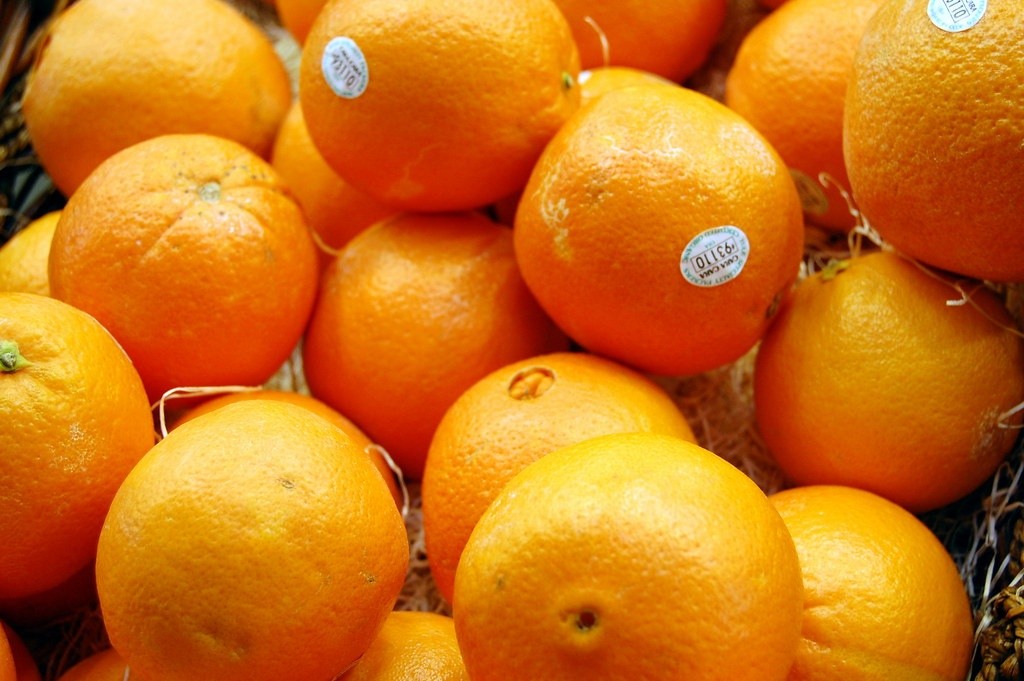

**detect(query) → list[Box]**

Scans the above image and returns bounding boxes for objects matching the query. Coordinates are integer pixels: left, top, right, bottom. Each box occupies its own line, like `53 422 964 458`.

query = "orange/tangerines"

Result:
0 0 1024 681
